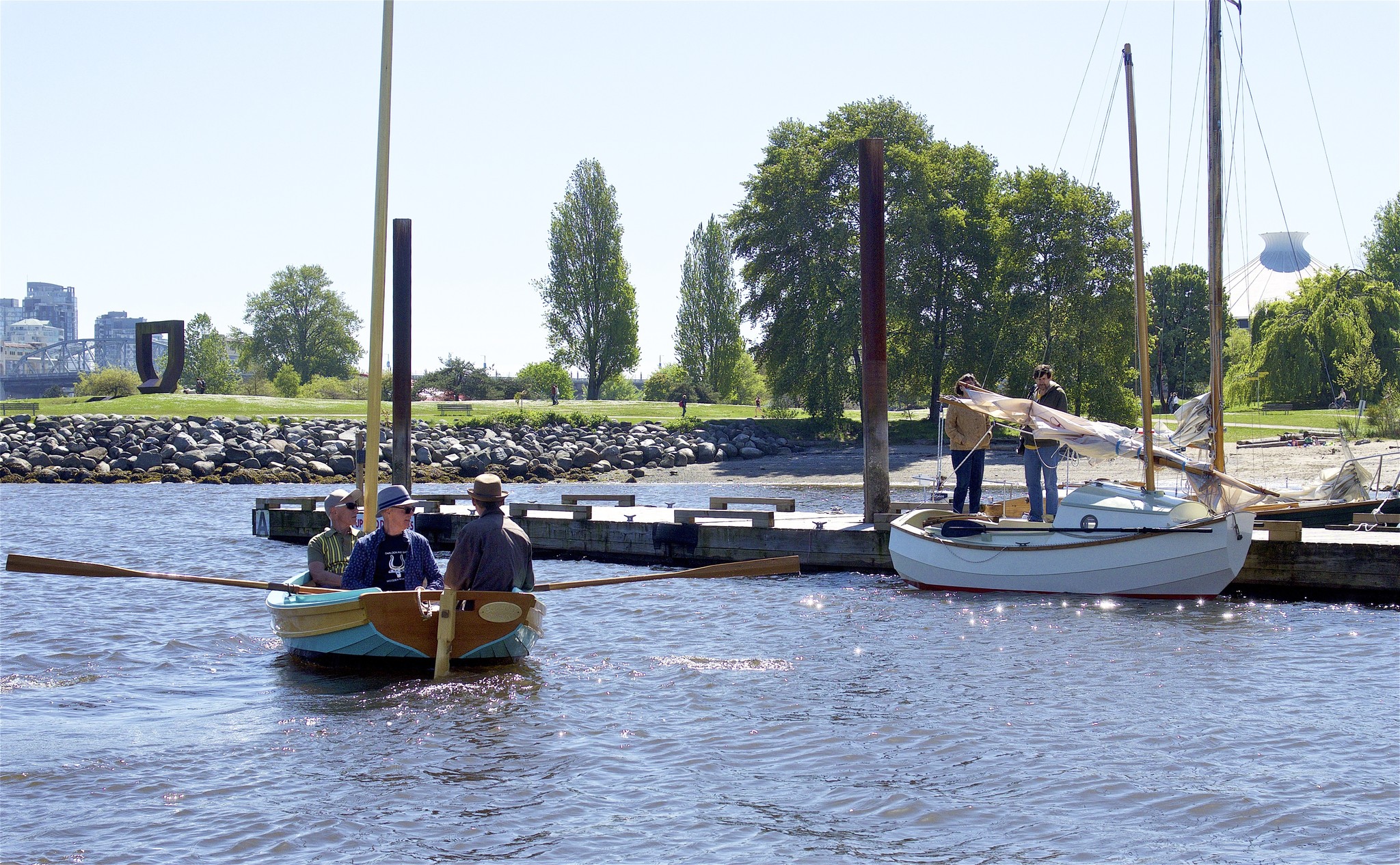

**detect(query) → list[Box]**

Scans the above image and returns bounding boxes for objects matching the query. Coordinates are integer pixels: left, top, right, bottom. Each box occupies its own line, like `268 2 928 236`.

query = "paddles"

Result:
533 555 800 595
5 553 347 594
941 519 1213 538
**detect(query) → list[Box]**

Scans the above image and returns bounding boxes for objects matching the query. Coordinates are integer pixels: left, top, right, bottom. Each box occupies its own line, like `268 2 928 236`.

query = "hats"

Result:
324 489 361 516
375 485 419 518
466 474 509 502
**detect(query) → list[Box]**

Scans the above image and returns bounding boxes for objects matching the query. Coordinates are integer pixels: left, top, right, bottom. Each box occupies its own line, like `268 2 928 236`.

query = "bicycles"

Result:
1328 397 1351 410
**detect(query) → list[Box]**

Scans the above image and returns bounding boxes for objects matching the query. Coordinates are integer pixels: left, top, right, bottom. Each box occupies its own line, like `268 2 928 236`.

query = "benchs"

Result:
256 495 326 511
0 403 39 416
709 497 795 512
1261 404 1293 415
873 513 901 531
891 502 983 513
509 502 592 520
561 494 635 507
1252 520 1302 542
412 494 505 513
1353 512 1400 525
674 509 774 527
437 404 473 416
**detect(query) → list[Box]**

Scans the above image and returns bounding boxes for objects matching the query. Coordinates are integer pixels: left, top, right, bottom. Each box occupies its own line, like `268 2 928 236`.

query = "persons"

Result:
681 395 687 416
307 489 370 590
341 485 444 604
196 377 205 394
1168 392 1178 414
1150 391 1155 414
551 383 560 405
1020 364 1068 522
944 374 993 513
1305 433 1320 446
754 395 764 418
444 474 535 611
1336 388 1346 409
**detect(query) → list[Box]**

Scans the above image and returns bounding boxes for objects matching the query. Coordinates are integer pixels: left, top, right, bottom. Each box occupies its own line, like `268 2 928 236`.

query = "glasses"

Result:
335 502 357 509
391 506 416 514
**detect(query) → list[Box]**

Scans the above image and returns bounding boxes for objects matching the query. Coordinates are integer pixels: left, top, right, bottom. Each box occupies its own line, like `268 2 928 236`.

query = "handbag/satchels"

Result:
1289 439 1298 446
1015 435 1025 456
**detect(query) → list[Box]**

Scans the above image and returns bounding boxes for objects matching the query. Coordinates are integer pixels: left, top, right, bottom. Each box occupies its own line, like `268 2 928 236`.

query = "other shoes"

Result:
1026 516 1043 522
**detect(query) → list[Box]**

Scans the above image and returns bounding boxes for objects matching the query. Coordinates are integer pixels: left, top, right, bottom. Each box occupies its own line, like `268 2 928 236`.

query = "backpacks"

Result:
679 399 684 407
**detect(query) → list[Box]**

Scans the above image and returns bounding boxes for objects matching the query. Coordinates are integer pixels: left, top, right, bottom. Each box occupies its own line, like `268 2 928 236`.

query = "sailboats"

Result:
1056 3 1400 533
261 1 545 685
881 1 1284 604
